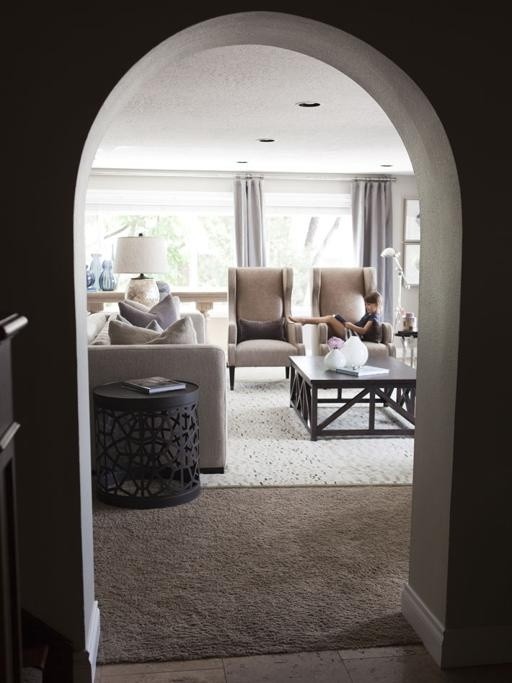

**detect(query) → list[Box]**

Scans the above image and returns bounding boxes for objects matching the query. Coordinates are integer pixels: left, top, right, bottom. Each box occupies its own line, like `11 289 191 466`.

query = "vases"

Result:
85 253 116 291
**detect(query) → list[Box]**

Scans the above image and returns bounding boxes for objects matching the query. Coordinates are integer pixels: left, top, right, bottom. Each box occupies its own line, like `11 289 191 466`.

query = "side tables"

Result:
92 378 199 508
395 331 417 367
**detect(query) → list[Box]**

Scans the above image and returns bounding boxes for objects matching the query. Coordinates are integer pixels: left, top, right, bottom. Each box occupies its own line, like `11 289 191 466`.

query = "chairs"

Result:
227 266 305 390
311 267 395 357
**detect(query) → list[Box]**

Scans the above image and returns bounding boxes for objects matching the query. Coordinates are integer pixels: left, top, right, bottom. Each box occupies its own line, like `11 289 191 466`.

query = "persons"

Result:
288 290 383 341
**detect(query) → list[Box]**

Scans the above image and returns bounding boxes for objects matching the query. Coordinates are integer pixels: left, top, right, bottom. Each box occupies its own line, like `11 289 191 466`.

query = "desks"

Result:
87 292 227 319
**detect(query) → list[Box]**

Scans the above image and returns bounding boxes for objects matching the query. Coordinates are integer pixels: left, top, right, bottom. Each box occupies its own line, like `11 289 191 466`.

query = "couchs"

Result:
88 307 227 474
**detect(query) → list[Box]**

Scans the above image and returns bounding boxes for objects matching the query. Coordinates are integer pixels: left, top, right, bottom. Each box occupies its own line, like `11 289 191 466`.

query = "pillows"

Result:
147 295 180 330
364 324 383 343
143 320 164 333
237 318 285 342
108 320 161 344
115 313 134 326
117 301 161 330
123 298 150 313
141 316 198 345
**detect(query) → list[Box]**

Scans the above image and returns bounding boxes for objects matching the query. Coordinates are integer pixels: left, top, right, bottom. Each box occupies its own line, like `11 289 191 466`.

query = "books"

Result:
335 365 390 377
121 375 186 395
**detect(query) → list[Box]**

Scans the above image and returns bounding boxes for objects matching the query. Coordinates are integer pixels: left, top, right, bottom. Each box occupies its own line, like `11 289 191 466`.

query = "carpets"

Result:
90 484 423 665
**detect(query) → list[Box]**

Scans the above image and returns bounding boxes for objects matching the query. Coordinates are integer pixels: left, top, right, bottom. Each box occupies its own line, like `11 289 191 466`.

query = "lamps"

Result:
380 247 419 290
114 234 168 309
